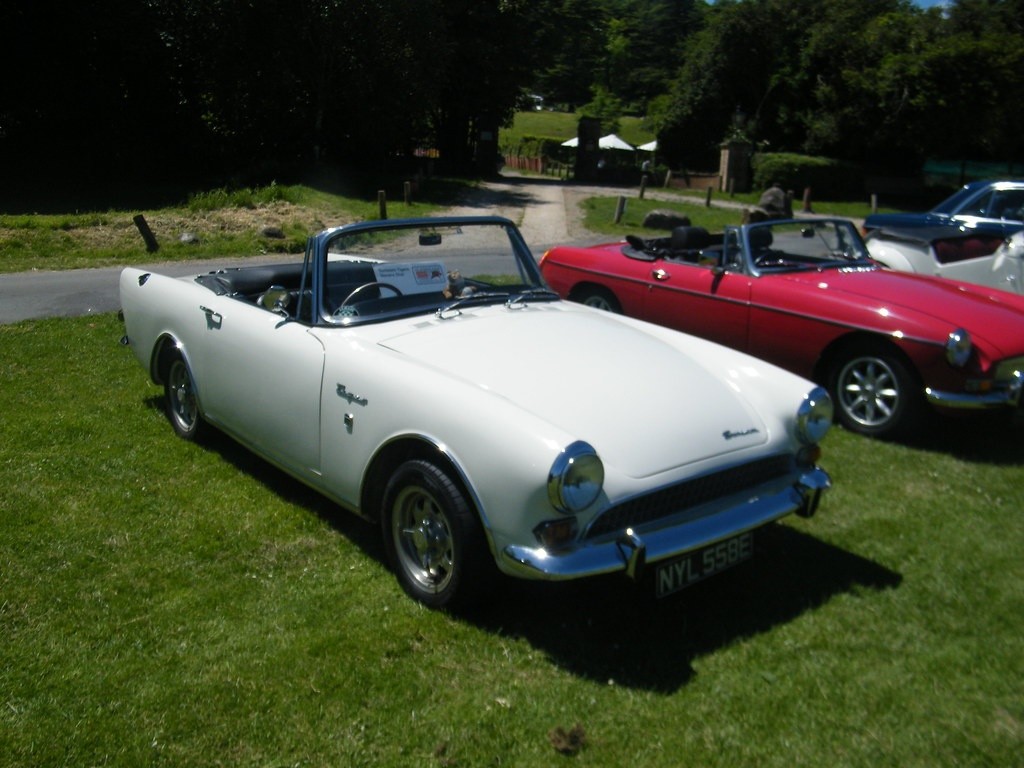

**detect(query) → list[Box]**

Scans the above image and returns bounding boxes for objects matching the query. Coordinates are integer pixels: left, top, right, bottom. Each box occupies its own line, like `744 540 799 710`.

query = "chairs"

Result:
666 223 732 269
232 259 379 322
735 229 794 266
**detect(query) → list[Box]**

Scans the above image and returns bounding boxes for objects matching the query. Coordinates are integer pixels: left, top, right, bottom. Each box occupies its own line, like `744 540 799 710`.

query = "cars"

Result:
865 222 1023 296
119 213 834 610
862 179 1023 238
538 216 1023 438
